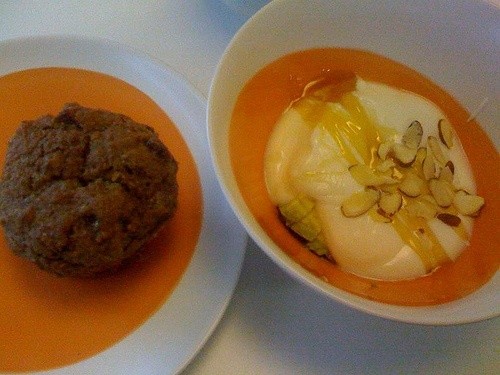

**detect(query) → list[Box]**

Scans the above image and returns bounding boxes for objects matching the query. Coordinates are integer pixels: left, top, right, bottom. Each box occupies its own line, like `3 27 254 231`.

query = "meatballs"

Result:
1 103 180 280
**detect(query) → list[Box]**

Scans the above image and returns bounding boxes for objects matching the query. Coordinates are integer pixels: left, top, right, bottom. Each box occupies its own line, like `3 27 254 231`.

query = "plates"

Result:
207 0 499 326
0 34 249 375
